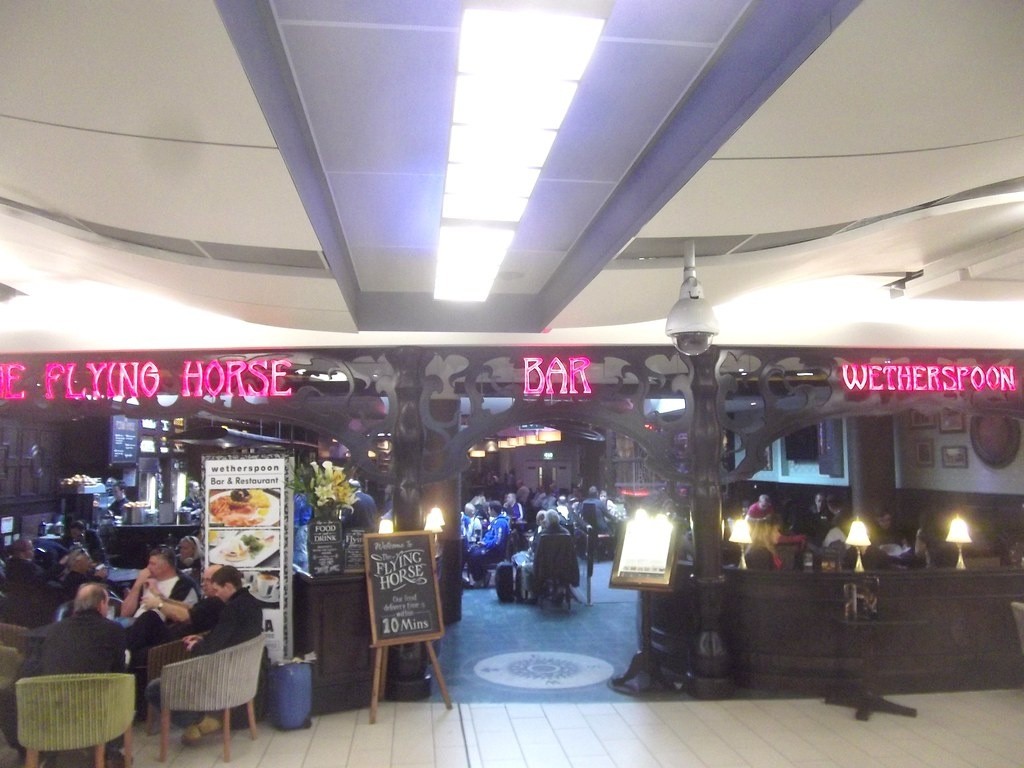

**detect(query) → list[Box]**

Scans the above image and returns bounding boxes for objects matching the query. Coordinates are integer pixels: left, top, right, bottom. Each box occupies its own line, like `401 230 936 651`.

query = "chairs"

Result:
533 532 577 614
482 528 512 571
0 624 266 768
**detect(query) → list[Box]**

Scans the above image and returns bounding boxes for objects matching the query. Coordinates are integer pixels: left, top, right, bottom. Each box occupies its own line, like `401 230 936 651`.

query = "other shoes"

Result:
180 715 221 744
471 572 490 588
104 750 133 768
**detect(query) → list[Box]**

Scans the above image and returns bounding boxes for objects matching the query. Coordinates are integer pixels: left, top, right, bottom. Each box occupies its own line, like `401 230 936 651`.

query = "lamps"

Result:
728 515 753 569
378 505 446 553
470 427 562 458
944 513 972 570
845 516 872 572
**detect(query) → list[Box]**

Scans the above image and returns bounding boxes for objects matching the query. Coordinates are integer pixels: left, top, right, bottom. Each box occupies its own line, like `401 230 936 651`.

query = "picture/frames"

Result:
939 410 966 434
941 446 969 468
909 409 936 430
970 414 1021 468
914 438 935 468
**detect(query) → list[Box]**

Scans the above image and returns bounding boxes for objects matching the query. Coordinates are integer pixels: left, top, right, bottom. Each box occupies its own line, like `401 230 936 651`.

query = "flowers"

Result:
280 455 361 515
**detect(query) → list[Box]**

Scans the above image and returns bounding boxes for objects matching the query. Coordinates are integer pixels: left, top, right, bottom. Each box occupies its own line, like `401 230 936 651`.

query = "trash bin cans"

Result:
270 662 312 730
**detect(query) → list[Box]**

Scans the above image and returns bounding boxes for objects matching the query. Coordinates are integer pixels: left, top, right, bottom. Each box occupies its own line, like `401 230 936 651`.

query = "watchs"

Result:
157 602 164 610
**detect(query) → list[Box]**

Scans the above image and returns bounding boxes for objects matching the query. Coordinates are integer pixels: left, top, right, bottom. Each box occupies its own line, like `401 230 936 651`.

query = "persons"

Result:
182 481 203 521
744 492 1024 572
460 468 694 602
292 466 395 573
108 480 131 518
3 521 263 768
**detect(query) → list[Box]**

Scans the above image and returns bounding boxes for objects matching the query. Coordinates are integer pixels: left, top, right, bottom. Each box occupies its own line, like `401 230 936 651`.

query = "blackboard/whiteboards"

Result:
365 533 445 638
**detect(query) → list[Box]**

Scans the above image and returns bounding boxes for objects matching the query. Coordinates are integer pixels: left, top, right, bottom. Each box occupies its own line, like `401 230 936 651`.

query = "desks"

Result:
825 608 930 719
512 521 528 529
107 568 142 594
